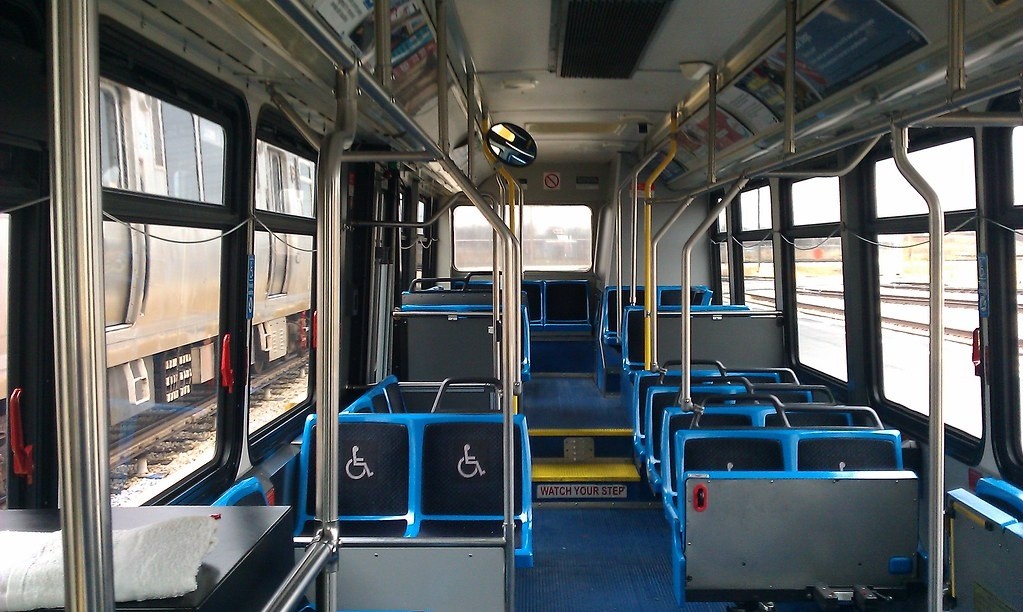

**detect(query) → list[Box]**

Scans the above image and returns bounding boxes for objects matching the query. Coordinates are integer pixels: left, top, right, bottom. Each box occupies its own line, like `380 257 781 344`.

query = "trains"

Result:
1 78 318 451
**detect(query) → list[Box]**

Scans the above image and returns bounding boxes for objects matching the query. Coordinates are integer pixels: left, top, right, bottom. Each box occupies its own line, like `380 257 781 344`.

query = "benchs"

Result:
599 283 907 604
206 278 591 612
